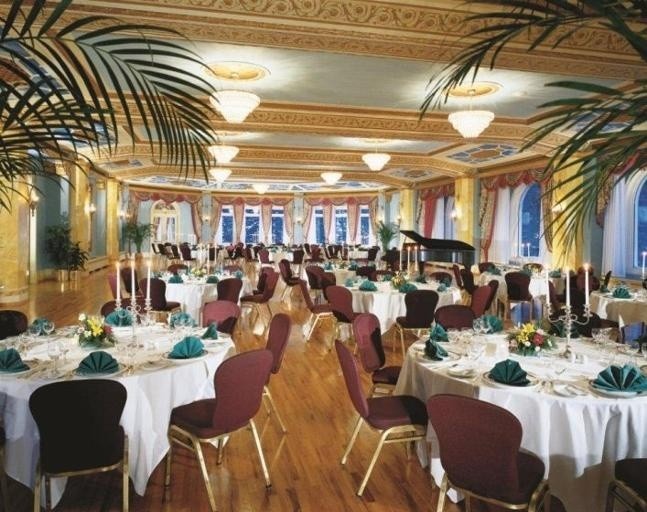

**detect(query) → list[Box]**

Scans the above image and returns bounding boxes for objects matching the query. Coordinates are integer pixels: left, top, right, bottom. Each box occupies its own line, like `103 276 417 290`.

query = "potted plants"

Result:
45 222 89 282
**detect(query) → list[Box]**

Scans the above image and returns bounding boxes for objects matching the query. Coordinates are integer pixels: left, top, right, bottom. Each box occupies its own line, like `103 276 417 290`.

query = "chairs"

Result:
164 349 274 512
334 339 437 497
0 310 27 340
426 393 550 512
605 458 647 511
29 378 130 512
261 313 293 435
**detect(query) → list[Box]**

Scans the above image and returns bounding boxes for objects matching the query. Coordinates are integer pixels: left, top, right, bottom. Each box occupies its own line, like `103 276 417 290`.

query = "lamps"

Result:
201 60 270 125
30 189 38 216
361 138 391 172
208 131 343 196
90 197 96 223
444 80 504 139
551 194 564 221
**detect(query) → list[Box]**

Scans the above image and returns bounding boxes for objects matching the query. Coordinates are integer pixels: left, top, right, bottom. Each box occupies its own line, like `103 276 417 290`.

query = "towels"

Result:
30 318 55 337
76 351 118 375
0 348 29 373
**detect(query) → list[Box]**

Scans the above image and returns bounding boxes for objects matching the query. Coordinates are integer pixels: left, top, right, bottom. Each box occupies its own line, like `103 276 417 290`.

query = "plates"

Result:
72 362 128 379
480 371 541 390
415 351 462 364
202 333 231 349
0 360 40 377
161 349 209 364
588 378 638 396
554 384 587 396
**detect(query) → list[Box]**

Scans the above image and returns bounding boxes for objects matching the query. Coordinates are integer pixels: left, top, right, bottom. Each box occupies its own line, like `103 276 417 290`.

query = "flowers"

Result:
77 313 118 349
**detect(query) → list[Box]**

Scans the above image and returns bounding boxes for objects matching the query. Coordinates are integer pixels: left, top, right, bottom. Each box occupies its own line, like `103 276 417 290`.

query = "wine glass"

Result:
18 320 70 379
447 318 490 358
625 340 647 363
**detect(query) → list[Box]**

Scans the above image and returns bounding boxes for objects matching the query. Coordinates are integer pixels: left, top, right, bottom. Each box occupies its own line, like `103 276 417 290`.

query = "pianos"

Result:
398 229 476 268
